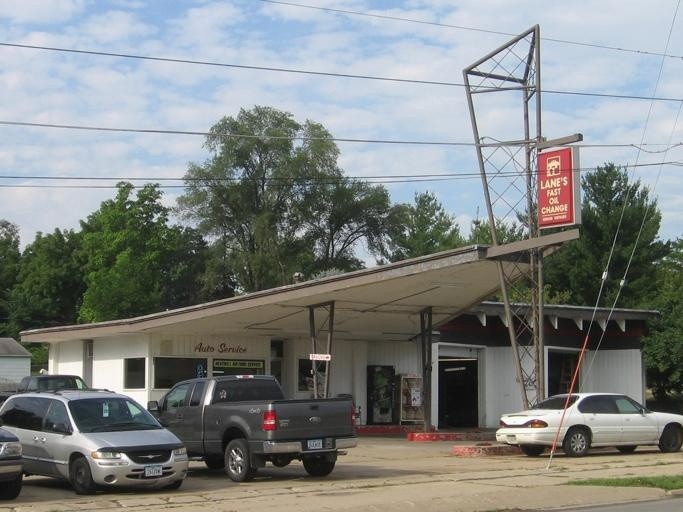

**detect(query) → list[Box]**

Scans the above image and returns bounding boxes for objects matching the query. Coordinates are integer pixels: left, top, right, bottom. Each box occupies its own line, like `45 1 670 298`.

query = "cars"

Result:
495 392 683 456
0 427 24 500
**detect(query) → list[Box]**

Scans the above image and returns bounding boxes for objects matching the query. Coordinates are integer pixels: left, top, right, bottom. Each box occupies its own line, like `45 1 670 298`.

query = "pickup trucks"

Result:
131 375 357 483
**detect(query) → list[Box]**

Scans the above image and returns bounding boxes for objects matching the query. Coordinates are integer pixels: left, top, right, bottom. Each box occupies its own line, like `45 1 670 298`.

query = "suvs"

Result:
16 375 108 394
0 389 189 489
